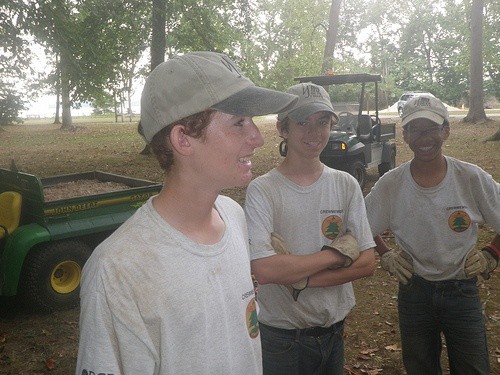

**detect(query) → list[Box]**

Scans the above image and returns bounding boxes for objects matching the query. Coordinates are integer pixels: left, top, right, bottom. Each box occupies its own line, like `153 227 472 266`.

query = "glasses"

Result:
406 123 446 134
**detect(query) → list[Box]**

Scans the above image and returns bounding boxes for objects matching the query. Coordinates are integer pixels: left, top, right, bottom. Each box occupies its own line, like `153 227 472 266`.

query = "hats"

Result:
140 51 298 144
400 92 448 128
278 82 340 124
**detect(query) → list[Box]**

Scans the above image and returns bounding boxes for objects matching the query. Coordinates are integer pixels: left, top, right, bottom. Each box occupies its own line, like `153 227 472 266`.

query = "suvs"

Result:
397 91 446 117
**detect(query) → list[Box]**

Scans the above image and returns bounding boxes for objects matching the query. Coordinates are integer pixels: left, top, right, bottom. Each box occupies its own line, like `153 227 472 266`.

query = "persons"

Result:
243 83 377 375
75 50 299 375
365 93 500 375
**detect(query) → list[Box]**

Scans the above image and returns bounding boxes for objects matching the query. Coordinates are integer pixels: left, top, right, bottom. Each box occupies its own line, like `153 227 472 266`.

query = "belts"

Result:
259 319 344 336
